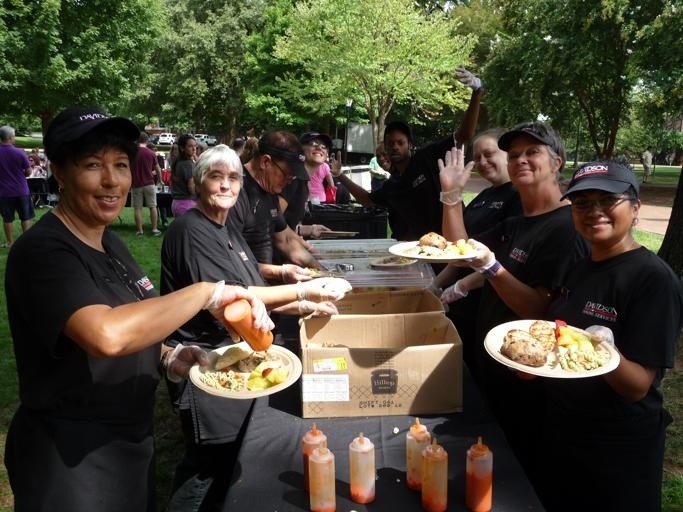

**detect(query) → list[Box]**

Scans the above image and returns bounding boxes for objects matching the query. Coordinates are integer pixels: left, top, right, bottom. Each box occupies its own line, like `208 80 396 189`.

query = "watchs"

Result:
479 261 503 281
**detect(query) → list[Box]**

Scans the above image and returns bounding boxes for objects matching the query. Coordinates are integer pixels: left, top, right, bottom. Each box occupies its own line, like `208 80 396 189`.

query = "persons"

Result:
640 146 653 184
4 103 277 512
329 64 483 245
227 130 361 285
164 144 351 497
447 161 680 511
427 126 521 331
436 122 592 367
0 126 257 247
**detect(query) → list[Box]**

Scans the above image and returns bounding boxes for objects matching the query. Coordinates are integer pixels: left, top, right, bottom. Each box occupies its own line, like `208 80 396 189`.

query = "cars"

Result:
149 132 217 146
152 151 172 194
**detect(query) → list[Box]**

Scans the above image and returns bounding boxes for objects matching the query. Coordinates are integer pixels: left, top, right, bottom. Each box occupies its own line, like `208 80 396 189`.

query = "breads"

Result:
500 319 556 367
419 232 448 248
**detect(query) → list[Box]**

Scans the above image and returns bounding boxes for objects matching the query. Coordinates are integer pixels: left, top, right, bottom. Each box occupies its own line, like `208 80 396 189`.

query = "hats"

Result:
259 139 310 182
301 132 332 145
44 110 141 164
496 128 559 154
560 162 641 201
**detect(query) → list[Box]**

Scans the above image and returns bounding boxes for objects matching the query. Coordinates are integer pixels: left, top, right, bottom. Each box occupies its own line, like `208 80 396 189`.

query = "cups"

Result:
163 186 169 193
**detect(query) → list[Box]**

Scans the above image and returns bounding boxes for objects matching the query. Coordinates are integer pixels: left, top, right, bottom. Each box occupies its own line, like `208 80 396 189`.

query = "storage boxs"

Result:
295 286 445 330
298 237 437 292
296 312 465 419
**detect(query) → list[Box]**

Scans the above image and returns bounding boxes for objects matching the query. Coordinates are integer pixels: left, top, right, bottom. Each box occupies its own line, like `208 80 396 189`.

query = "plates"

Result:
482 319 622 380
189 342 304 401
319 230 360 239
310 270 348 278
387 240 479 262
366 256 418 267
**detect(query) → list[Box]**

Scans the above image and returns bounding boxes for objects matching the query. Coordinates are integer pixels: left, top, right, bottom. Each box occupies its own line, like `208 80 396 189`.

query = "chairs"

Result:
26 176 56 208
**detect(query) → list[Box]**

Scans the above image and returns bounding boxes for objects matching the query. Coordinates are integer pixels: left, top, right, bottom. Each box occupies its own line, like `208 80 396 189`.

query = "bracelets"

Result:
439 190 462 207
159 346 175 372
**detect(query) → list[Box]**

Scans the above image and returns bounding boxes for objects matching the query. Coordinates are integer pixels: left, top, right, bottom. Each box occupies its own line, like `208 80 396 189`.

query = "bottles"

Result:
462 433 494 511
305 442 337 512
419 435 449 512
301 421 328 495
347 430 378 506
404 417 433 488
221 298 276 353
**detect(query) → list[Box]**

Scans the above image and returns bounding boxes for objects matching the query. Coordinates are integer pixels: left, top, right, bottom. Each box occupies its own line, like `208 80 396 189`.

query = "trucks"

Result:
330 122 388 164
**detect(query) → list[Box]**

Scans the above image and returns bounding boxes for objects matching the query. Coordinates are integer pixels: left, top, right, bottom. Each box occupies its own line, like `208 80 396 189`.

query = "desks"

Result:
222 327 546 510
120 186 173 226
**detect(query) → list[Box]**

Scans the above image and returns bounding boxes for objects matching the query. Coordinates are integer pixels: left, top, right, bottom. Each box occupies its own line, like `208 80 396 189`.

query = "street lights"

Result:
341 87 354 167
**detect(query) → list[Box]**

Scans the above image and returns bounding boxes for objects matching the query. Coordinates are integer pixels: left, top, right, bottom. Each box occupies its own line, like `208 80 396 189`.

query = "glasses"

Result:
308 140 329 149
270 158 297 182
573 195 634 209
